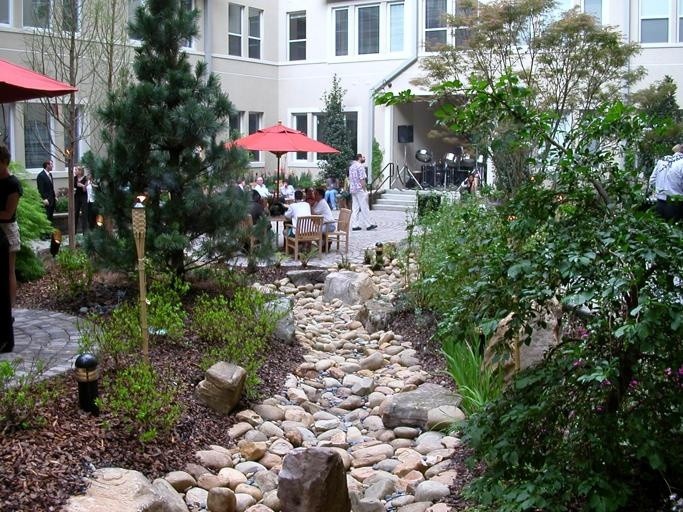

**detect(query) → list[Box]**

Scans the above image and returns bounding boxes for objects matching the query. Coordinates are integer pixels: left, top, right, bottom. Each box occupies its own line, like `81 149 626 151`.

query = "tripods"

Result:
391 144 423 190
457 149 487 190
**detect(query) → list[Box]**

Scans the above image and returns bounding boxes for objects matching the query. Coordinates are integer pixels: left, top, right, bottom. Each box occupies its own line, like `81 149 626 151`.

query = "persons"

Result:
74 166 88 235
466 174 476 193
236 173 350 253
0 145 24 308
648 144 683 222
349 153 378 231
37 159 55 224
82 169 99 231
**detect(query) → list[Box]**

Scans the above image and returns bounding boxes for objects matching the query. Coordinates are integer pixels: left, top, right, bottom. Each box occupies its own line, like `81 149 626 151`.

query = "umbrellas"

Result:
226 120 341 200
0 58 78 106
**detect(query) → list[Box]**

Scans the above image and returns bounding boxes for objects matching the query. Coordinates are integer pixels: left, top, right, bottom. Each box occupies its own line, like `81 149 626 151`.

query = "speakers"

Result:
398 125 413 143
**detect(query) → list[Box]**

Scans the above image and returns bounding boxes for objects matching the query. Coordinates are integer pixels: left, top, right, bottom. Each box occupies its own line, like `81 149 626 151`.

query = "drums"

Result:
441 152 459 167
460 153 475 168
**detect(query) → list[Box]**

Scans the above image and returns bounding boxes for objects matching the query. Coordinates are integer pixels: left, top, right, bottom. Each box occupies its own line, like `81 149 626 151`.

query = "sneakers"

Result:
366 224 378 231
352 227 362 231
333 207 336 211
322 241 332 252
0 342 15 354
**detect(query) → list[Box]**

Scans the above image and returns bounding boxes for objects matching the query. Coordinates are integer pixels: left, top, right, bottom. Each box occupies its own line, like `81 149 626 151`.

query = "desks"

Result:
239 213 262 257
266 216 291 248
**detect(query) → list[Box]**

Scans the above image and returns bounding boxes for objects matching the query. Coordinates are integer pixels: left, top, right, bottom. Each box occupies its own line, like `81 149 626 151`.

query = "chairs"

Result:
325 207 352 253
285 215 324 262
